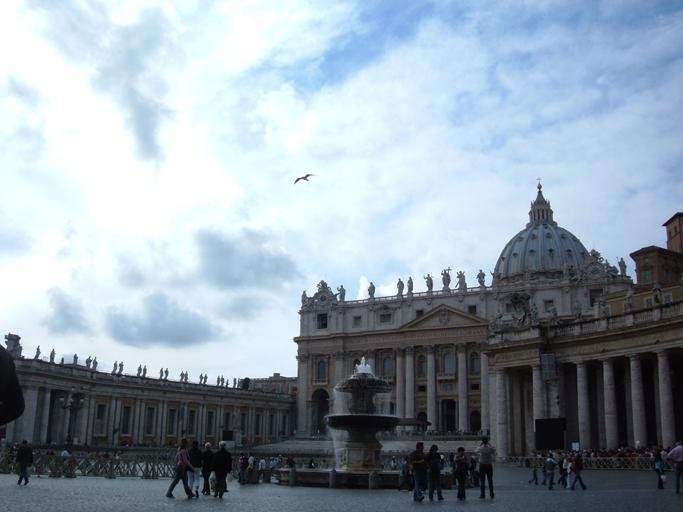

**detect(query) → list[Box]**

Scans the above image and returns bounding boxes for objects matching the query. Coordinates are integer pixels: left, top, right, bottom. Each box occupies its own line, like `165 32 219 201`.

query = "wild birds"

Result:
294 174 315 184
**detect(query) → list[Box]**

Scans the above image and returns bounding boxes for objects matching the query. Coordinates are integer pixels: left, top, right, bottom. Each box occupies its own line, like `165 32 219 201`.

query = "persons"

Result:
529 441 683 494
166 437 296 500
12 440 73 486
301 269 501 306
391 436 496 502
618 257 627 275
